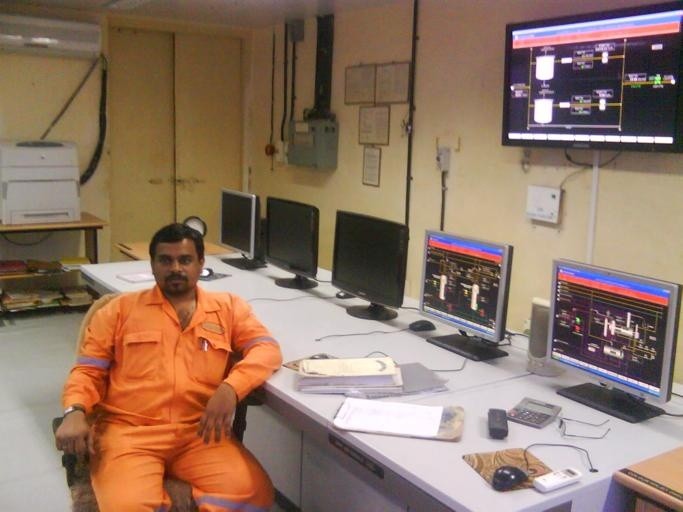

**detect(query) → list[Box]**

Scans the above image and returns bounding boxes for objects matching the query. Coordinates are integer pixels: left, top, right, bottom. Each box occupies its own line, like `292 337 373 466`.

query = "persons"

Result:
54 223 285 509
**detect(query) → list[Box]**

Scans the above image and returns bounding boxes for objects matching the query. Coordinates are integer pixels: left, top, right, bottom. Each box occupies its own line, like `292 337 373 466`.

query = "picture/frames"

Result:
371 59 411 108
353 102 392 148
343 61 375 107
359 144 384 187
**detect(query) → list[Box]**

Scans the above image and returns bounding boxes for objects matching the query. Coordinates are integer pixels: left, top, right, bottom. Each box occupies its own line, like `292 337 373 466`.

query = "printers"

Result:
0 141 80 224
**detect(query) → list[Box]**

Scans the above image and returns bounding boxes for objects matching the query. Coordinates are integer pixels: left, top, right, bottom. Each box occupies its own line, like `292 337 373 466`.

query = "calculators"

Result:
507 397 561 428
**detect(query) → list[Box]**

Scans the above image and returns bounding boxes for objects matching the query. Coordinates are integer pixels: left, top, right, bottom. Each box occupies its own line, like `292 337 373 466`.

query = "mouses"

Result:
409 320 435 331
336 291 356 298
493 466 527 491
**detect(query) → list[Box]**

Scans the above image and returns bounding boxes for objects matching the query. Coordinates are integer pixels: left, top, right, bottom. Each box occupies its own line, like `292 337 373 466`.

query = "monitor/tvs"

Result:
219 188 267 270
502 0 683 153
546 258 683 424
331 210 408 321
419 229 513 361
265 196 320 289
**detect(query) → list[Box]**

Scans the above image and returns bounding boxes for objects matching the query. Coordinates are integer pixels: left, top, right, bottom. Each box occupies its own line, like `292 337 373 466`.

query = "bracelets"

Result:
63 406 86 419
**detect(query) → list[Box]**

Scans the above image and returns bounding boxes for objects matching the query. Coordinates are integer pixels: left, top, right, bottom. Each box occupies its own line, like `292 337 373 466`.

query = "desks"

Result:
612 443 682 512
69 254 682 512
116 235 238 261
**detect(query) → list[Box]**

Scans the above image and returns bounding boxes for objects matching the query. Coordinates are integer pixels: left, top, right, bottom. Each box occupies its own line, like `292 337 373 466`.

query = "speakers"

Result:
526 297 565 376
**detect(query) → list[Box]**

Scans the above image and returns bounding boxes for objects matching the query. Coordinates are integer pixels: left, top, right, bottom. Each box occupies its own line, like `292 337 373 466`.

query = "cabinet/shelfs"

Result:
0 212 108 325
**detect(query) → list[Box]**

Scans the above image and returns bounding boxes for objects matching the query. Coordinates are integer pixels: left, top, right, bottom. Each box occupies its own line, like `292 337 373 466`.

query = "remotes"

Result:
533 468 582 493
488 408 508 440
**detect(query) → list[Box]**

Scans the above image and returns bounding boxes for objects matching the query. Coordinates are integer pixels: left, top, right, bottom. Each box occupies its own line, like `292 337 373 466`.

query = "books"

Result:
300 367 404 393
297 357 394 386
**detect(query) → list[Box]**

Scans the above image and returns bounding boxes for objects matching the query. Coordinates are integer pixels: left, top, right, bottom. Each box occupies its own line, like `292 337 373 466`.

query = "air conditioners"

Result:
0 12 104 64
